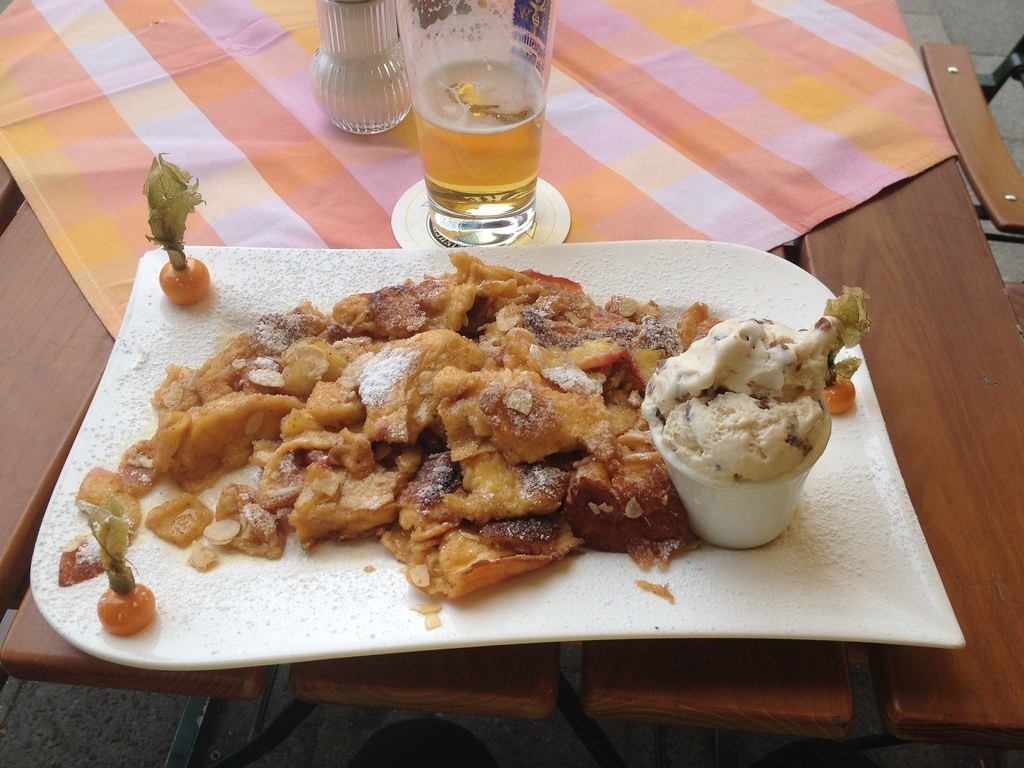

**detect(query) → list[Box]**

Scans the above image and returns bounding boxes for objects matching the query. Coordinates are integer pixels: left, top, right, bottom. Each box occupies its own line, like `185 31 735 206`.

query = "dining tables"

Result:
0 0 1024 768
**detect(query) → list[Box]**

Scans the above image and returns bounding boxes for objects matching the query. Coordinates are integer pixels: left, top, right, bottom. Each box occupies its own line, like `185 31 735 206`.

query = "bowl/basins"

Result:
650 401 831 547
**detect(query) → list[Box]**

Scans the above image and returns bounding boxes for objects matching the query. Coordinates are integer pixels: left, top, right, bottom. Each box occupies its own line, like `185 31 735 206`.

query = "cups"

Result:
395 0 558 247
312 0 411 135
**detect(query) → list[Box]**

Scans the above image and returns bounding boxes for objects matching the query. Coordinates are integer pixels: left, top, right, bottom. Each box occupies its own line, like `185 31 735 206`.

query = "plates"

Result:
30 239 965 670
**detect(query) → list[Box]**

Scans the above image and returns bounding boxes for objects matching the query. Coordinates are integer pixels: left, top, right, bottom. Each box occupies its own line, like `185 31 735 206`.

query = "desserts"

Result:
647 318 832 486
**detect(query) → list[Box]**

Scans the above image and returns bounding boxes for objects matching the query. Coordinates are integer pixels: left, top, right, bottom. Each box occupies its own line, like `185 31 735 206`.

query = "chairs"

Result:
918 34 1024 245
